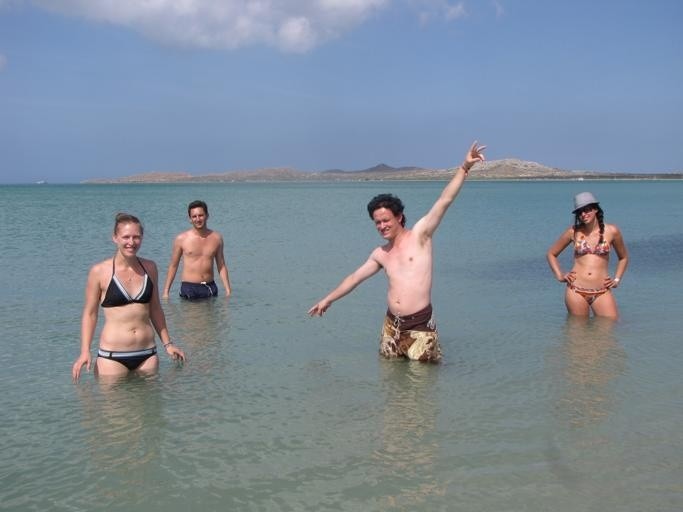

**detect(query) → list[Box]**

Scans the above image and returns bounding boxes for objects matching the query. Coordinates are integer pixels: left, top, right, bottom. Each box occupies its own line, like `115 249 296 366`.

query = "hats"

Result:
572 192 600 214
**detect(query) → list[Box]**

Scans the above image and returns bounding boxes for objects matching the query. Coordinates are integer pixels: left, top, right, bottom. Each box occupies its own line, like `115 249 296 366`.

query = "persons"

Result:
71 212 187 378
306 139 487 363
545 192 628 318
162 199 231 301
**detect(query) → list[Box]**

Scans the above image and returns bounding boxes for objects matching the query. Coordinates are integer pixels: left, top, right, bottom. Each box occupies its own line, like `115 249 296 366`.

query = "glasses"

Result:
577 206 592 215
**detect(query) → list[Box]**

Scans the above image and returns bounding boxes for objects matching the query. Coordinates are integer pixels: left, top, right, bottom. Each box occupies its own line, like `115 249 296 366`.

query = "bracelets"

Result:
461 164 470 176
163 342 173 347
614 278 620 282
